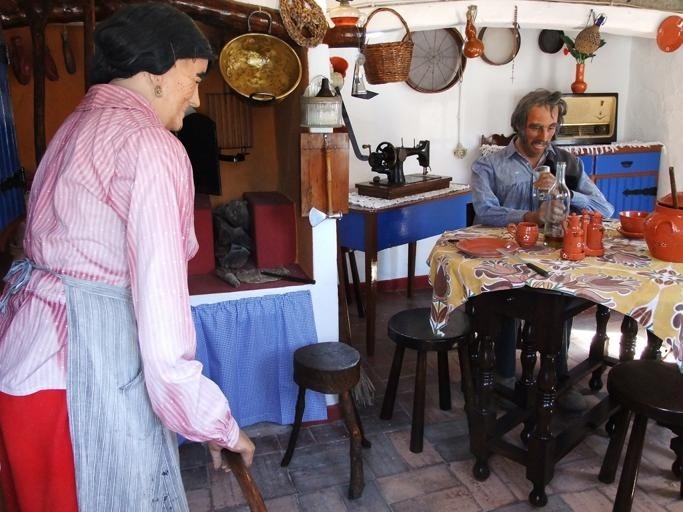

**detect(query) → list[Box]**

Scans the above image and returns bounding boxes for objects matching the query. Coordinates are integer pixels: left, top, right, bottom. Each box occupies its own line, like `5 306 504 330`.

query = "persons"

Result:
470 88 615 421
0 7 255 512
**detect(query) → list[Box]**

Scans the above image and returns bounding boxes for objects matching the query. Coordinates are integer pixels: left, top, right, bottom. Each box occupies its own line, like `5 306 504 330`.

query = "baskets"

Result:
360 7 414 85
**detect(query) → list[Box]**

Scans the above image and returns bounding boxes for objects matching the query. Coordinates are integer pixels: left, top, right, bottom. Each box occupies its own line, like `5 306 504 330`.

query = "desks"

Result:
438 217 683 507
337 184 472 355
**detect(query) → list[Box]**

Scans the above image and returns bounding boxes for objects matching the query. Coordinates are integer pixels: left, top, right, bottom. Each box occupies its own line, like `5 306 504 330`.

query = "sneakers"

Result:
493 373 519 411
556 384 586 412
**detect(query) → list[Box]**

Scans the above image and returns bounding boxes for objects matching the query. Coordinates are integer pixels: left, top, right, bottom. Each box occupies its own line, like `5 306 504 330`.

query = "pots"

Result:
218 10 303 105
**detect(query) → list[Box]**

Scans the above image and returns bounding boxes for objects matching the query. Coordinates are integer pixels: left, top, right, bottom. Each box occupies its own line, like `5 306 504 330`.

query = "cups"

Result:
533 165 553 199
507 221 539 248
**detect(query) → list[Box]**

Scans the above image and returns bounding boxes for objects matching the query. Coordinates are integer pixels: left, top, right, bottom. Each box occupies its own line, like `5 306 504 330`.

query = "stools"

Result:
379 307 479 455
596 358 682 512
337 250 363 344
281 340 371 501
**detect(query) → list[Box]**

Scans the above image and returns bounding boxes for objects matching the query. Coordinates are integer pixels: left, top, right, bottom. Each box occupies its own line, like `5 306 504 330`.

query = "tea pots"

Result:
558 209 606 261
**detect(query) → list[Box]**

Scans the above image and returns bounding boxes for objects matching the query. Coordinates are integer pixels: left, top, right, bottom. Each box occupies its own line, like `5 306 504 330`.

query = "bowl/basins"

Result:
618 210 653 232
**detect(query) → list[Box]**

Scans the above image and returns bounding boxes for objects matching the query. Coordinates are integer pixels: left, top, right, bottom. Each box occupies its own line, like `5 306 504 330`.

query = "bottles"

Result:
644 191 682 262
542 161 572 248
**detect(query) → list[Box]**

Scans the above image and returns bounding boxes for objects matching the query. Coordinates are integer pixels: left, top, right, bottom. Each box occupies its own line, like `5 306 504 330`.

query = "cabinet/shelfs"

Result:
555 140 665 213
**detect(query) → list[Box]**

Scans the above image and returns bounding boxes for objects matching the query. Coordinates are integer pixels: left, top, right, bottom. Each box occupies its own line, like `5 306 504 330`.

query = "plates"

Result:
656 14 683 53
456 238 519 258
616 226 644 240
